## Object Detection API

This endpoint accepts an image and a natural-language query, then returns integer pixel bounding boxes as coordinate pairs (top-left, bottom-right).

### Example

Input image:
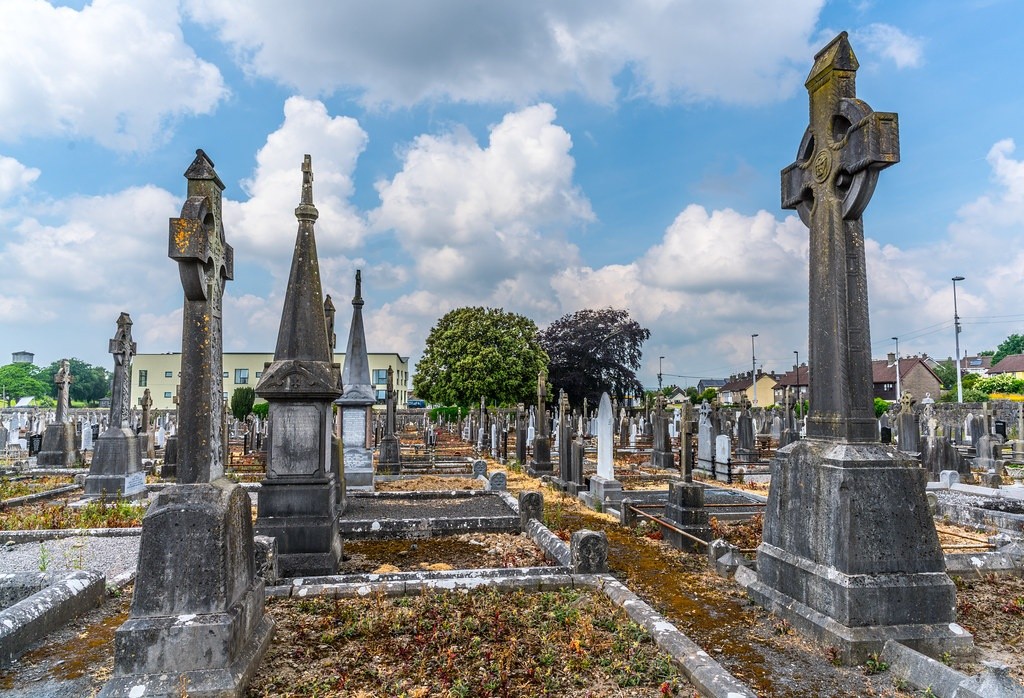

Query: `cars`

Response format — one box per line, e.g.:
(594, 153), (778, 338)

(406, 400), (426, 408)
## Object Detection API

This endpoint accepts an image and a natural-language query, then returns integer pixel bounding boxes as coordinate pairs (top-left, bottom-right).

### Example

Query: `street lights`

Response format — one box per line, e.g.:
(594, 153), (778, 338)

(891, 336), (905, 404)
(751, 333), (761, 407)
(792, 350), (803, 401)
(951, 276), (968, 403)
(656, 355), (664, 389)
